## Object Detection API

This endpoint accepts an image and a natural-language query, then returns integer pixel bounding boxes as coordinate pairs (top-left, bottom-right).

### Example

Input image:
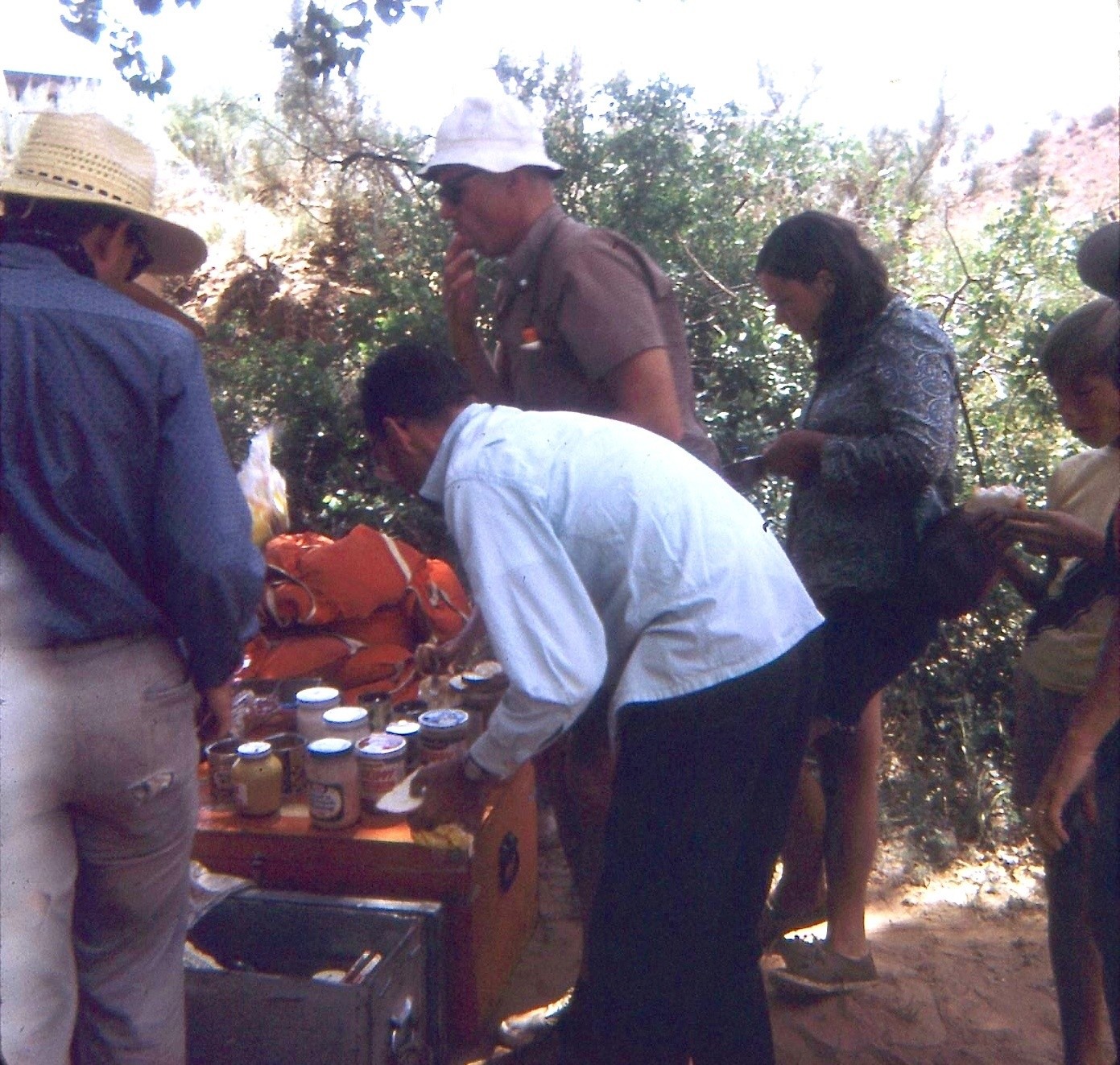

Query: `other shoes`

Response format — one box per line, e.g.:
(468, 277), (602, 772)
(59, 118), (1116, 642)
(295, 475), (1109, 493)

(497, 986), (576, 1049)
(756, 894), (826, 938)
(771, 935), (880, 992)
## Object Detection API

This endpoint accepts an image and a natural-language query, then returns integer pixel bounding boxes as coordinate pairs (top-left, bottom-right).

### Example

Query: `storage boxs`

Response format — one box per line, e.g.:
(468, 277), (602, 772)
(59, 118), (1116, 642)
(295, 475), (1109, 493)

(190, 744), (536, 1044)
(178, 886), (446, 1065)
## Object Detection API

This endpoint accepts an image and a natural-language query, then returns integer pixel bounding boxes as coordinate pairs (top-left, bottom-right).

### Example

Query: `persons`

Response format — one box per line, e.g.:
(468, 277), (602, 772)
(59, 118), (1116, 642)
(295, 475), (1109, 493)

(754, 210), (960, 993)
(1033, 222), (1120, 1051)
(417, 96), (727, 1050)
(970, 296), (1120, 1065)
(0, 110), (265, 1065)
(361, 341), (826, 1065)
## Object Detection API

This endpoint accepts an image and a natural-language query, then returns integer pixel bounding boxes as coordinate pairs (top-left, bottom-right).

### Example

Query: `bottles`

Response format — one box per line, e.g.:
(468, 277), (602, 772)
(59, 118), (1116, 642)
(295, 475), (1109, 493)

(519, 328), (546, 351)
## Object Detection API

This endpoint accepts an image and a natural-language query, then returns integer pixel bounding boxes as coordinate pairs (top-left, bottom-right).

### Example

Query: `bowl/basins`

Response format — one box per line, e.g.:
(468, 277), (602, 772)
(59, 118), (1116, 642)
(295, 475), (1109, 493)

(725, 455), (766, 485)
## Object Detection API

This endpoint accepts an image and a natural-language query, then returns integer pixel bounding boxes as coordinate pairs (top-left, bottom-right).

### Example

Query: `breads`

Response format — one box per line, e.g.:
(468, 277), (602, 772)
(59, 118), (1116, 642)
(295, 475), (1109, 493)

(970, 485), (1027, 508)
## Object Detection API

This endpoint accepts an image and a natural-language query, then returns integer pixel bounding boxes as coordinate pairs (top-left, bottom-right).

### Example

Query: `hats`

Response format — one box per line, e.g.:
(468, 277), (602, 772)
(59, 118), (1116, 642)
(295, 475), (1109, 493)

(416, 93), (563, 178)
(2, 111), (209, 276)
(1077, 222), (1120, 302)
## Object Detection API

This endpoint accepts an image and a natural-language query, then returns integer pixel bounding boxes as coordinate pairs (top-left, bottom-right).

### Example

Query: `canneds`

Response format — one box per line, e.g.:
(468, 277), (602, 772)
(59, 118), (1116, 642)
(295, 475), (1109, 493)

(228, 672), (503, 829)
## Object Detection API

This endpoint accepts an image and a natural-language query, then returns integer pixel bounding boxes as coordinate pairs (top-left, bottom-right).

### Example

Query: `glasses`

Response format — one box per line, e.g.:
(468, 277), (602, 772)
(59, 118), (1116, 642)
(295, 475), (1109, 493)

(131, 233), (154, 278)
(440, 169), (477, 204)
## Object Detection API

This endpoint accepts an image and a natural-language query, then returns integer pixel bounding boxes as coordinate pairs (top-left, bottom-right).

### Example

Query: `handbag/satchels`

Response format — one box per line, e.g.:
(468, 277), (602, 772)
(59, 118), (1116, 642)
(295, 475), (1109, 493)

(900, 504), (1006, 619)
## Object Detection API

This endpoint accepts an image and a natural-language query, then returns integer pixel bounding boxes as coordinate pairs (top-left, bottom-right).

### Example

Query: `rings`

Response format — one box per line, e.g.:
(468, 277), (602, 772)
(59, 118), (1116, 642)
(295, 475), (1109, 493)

(1038, 810), (1045, 814)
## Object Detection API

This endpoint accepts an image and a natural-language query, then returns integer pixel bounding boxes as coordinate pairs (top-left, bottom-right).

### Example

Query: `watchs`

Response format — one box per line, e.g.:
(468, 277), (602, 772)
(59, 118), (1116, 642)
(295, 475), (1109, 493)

(460, 751), (500, 784)
